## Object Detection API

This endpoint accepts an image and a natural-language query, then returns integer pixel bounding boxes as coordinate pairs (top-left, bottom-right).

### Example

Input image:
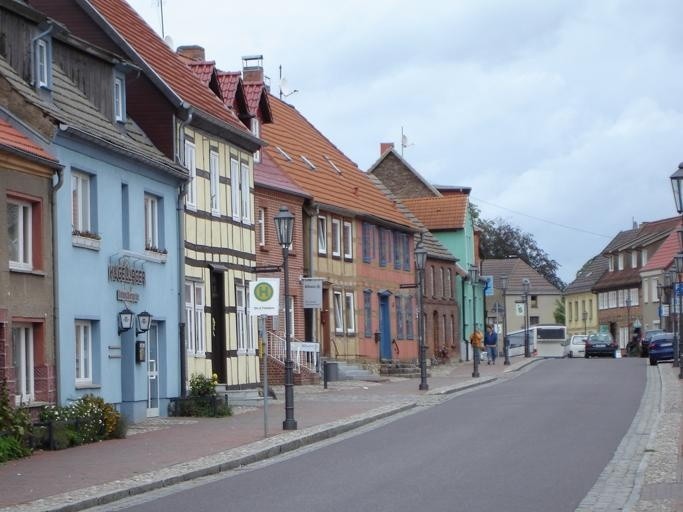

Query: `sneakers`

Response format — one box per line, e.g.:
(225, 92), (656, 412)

(488, 360), (495, 364)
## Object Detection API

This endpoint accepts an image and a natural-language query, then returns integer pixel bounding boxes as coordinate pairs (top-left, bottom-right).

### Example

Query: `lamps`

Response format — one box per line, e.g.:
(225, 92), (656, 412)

(117, 307), (153, 337)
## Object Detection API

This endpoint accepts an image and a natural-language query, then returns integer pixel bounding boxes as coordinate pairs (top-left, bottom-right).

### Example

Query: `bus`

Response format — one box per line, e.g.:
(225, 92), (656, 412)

(505, 325), (567, 359)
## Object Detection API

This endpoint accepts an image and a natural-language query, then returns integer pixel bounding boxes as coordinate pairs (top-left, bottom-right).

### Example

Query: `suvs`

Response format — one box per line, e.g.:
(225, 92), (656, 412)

(585, 333), (615, 358)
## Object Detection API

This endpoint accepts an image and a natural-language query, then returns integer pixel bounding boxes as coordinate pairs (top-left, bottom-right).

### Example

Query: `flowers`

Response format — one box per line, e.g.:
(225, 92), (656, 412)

(180, 373), (219, 397)
(39, 393), (121, 449)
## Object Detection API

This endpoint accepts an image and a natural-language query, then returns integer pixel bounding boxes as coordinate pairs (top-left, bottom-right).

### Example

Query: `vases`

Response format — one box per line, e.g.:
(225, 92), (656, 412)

(29, 419), (82, 451)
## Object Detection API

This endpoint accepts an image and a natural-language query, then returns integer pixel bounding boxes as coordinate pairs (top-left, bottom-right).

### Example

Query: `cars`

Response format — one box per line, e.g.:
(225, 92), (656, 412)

(566, 335), (594, 358)
(640, 329), (680, 365)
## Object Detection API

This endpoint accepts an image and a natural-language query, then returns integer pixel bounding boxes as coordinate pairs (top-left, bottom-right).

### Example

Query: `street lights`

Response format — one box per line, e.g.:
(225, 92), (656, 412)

(656, 282), (664, 329)
(500, 273), (510, 366)
(274, 207), (297, 430)
(626, 297), (632, 353)
(467, 264), (480, 378)
(664, 162), (683, 379)
(522, 278), (530, 357)
(413, 243), (429, 391)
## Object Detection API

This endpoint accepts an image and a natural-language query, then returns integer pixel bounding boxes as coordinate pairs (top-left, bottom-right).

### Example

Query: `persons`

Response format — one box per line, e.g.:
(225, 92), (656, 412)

(469, 328), (482, 363)
(484, 325), (498, 364)
